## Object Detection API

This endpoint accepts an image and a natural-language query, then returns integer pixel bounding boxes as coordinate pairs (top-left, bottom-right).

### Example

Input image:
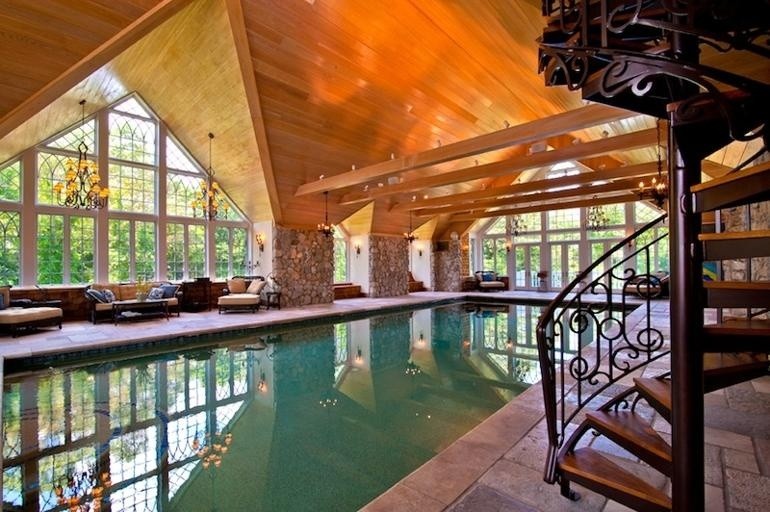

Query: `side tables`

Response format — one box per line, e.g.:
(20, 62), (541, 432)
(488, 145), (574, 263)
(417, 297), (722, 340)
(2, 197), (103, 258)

(268, 292), (281, 310)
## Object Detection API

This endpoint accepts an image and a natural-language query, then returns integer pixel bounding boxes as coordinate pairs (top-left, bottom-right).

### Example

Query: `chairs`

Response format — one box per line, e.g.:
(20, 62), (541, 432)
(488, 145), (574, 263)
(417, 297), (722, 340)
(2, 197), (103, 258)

(475, 271), (505, 291)
(624, 275), (670, 298)
(0, 285), (63, 337)
(218, 275), (267, 313)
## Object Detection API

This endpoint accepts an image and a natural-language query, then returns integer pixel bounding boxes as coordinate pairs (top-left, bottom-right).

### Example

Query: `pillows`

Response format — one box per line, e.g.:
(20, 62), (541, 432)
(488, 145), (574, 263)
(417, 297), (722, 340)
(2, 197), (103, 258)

(476, 272), (497, 280)
(86, 287), (115, 304)
(226, 278), (266, 294)
(150, 283), (182, 299)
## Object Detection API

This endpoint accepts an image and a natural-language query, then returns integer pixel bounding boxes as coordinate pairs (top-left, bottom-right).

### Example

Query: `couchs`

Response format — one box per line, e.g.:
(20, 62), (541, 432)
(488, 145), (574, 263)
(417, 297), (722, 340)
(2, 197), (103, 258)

(85, 283), (187, 324)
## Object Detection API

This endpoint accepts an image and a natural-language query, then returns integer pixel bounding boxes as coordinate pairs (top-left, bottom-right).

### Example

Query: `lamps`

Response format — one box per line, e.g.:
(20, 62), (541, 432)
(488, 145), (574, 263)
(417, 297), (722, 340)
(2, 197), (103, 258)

(638, 117), (670, 210)
(317, 191), (337, 238)
(190, 130), (230, 222)
(53, 95), (109, 209)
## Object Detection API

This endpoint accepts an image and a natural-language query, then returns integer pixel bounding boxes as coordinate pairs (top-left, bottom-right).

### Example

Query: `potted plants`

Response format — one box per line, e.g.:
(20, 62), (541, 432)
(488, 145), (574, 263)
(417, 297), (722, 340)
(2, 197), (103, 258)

(135, 283), (149, 300)
